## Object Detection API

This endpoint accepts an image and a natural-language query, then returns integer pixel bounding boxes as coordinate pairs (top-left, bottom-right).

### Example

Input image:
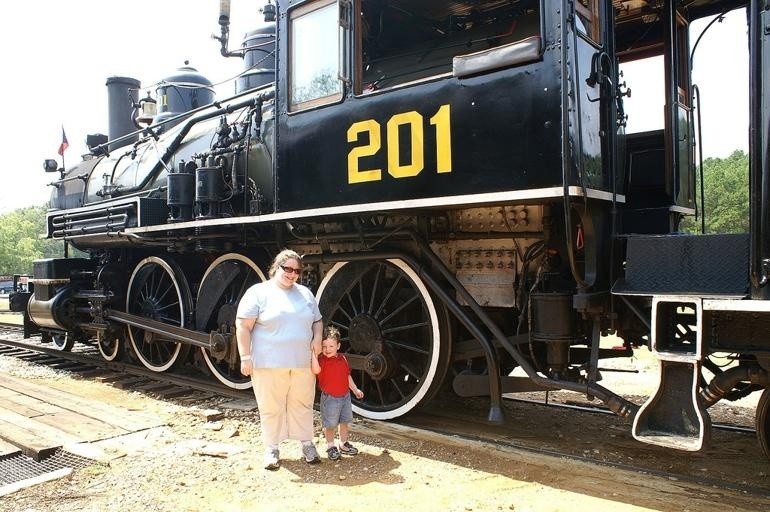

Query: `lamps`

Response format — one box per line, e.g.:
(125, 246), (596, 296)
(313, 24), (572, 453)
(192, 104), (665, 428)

(126, 89), (157, 136)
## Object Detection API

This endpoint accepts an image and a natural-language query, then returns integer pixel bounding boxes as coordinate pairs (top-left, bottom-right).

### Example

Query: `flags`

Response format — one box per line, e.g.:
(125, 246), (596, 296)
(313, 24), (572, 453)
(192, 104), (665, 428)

(57, 126), (71, 157)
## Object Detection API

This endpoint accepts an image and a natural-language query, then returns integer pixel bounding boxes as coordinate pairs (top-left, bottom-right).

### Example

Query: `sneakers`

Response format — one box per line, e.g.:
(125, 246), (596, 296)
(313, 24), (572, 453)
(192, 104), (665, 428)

(326, 446), (343, 461)
(339, 442), (359, 456)
(302, 443), (321, 464)
(264, 451), (280, 470)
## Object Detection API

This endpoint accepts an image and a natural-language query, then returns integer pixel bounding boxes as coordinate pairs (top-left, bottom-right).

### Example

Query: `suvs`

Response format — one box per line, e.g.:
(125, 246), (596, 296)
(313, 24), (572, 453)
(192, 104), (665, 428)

(0, 281), (14, 294)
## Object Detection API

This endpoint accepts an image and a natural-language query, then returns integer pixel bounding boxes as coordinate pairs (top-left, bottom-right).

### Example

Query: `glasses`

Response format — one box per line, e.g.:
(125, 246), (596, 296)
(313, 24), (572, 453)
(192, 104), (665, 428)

(279, 265), (302, 275)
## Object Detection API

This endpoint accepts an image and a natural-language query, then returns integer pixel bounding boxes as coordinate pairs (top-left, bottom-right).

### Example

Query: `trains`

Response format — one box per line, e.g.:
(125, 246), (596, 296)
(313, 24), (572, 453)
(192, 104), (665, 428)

(9, 1), (770, 462)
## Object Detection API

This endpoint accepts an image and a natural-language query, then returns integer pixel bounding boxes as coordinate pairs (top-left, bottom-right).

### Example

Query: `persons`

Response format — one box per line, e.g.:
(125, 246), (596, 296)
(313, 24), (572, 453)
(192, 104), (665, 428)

(233, 248), (325, 471)
(310, 322), (366, 462)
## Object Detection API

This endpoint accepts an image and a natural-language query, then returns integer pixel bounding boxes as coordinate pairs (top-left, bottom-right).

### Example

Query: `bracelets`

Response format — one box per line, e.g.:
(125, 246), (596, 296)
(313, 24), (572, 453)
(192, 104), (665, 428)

(239, 354), (252, 362)
